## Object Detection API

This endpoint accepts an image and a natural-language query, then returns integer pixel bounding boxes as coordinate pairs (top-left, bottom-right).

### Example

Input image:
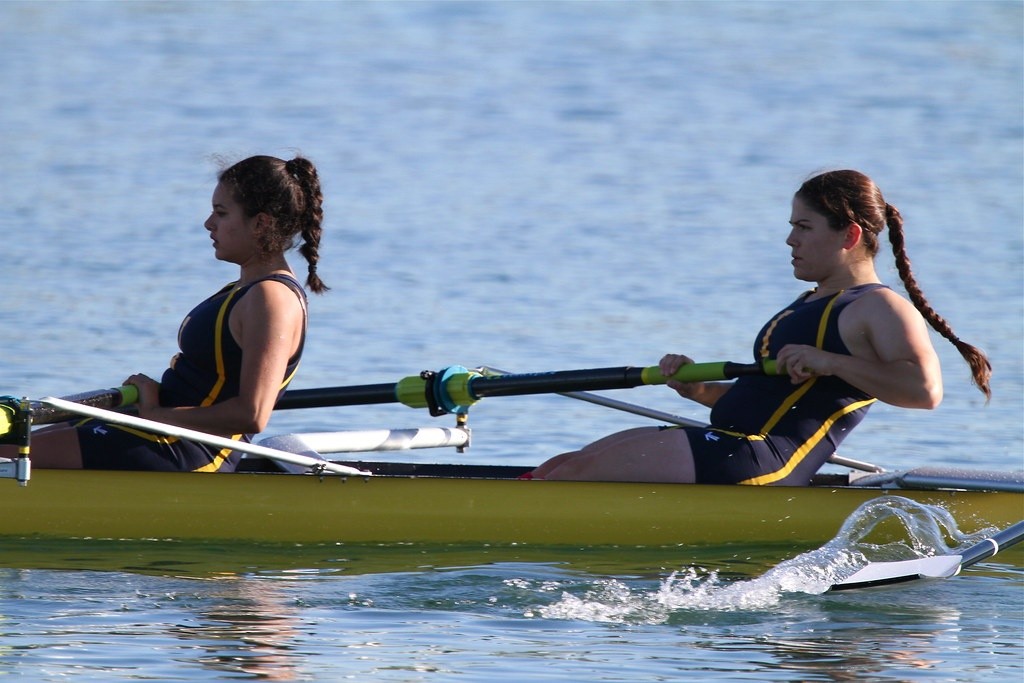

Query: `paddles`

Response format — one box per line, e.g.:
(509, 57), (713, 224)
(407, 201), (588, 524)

(0, 382), (140, 489)
(242, 422), (473, 477)
(825, 518), (1023, 592)
(271, 355), (805, 413)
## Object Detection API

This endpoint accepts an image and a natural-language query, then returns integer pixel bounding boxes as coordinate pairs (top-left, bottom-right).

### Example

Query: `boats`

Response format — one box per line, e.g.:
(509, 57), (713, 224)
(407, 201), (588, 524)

(0, 426), (1024, 574)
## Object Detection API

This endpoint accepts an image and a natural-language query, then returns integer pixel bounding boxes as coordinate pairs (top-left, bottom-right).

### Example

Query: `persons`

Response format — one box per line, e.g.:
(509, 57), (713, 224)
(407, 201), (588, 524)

(517, 169), (994, 489)
(0, 154), (330, 473)
